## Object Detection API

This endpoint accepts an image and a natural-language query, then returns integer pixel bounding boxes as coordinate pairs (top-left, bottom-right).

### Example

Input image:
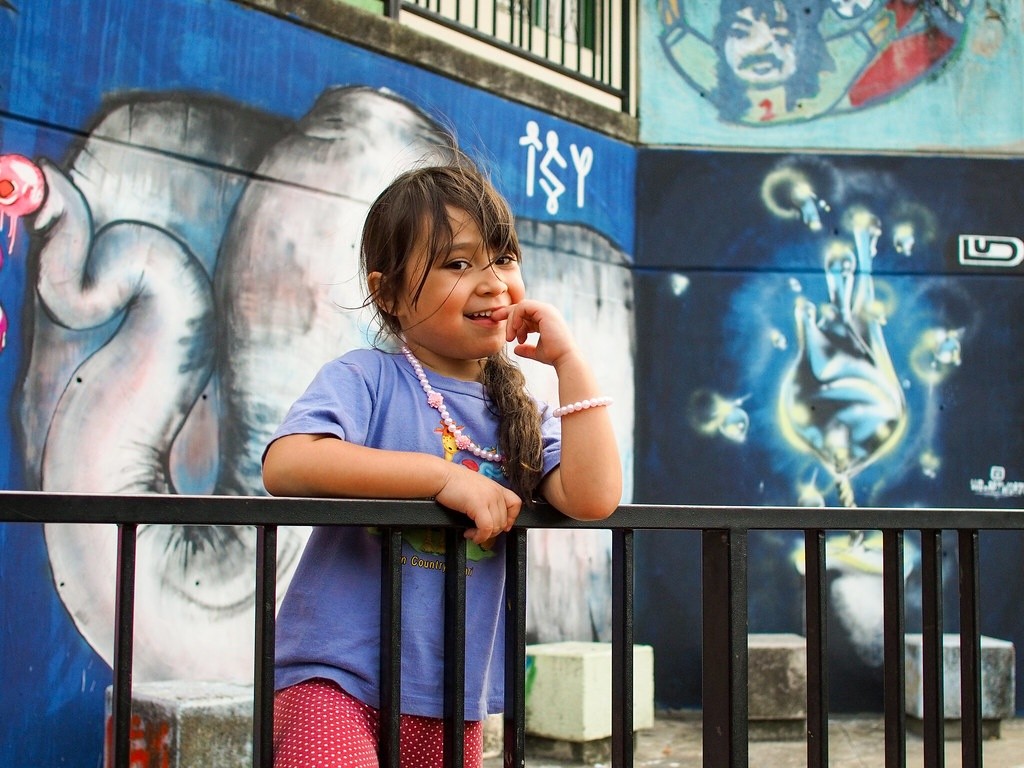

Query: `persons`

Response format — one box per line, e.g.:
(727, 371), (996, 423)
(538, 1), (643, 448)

(265, 164), (623, 768)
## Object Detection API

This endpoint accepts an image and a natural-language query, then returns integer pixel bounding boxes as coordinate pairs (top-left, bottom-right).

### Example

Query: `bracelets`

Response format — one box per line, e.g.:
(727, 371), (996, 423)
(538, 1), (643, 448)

(554, 396), (616, 417)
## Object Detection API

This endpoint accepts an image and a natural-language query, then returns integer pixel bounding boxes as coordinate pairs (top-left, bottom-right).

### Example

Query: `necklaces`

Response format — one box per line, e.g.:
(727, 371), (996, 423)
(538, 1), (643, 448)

(401, 348), (508, 462)
